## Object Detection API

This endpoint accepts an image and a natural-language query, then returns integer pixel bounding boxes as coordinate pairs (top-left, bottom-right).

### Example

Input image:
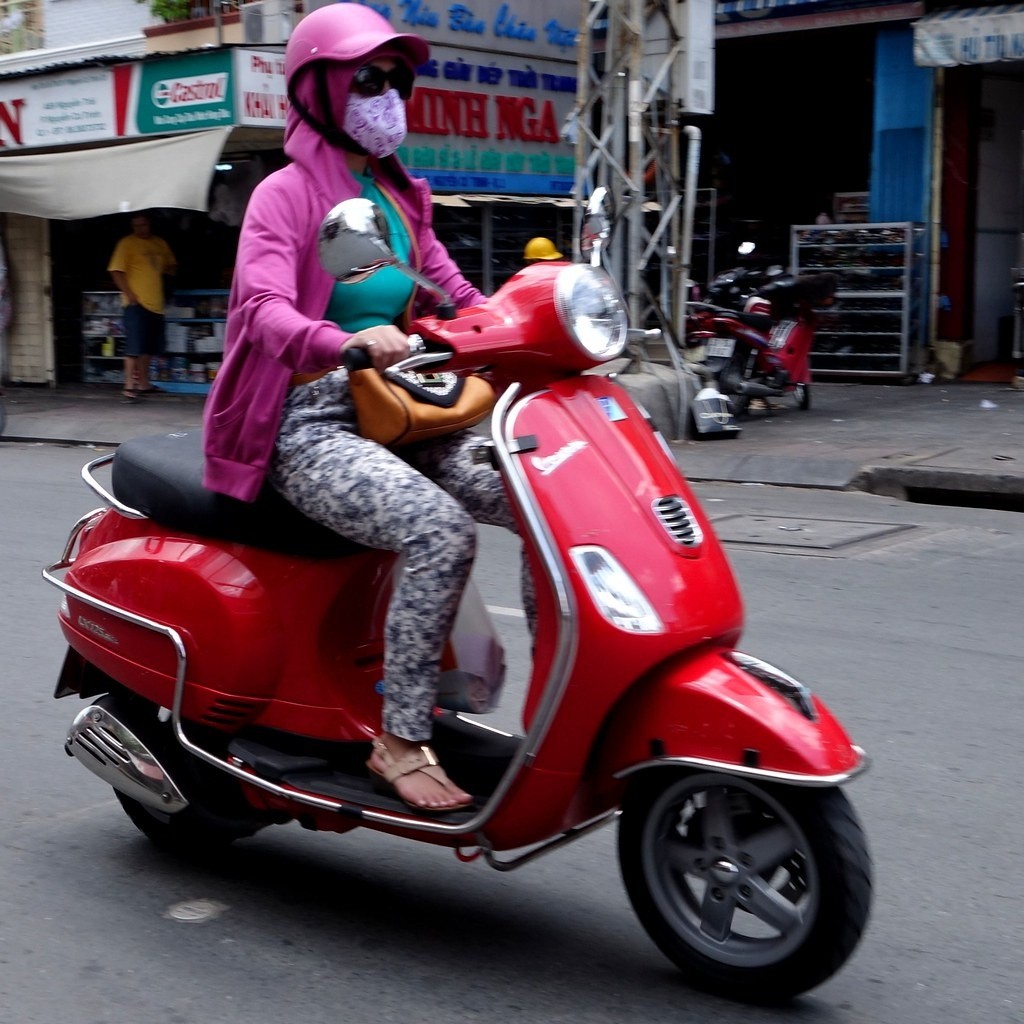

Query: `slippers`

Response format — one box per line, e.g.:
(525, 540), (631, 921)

(118, 389), (143, 404)
(137, 385), (167, 393)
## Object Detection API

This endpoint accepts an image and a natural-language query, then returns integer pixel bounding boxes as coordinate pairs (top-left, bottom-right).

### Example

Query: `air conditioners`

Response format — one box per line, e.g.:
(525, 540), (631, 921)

(240, 1), (290, 44)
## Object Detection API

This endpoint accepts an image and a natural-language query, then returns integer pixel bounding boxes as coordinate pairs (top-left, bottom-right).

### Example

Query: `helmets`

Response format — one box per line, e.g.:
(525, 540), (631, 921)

(284, 2), (430, 156)
(523, 237), (564, 261)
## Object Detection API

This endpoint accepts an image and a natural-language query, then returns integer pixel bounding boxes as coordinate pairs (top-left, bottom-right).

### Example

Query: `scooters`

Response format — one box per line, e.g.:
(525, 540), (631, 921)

(44, 185), (873, 1007)
(685, 239), (840, 418)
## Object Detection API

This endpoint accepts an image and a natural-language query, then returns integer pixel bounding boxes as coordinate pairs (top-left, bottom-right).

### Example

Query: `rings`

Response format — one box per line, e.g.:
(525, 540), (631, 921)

(366, 340), (378, 347)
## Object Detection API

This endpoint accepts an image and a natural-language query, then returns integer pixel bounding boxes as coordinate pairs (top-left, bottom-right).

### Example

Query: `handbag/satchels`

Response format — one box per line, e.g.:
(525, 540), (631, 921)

(347, 178), (498, 448)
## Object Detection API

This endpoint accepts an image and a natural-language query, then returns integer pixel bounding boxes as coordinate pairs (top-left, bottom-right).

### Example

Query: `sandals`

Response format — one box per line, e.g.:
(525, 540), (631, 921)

(365, 737), (477, 814)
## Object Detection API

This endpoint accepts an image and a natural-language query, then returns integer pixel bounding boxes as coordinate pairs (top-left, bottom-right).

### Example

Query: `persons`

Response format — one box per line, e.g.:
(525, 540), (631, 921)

(372, 206), (391, 253)
(107, 211), (178, 403)
(522, 236), (565, 267)
(204, 2), (537, 814)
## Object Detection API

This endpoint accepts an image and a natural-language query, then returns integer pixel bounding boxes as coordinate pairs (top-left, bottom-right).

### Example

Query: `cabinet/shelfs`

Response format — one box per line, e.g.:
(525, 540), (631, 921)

(80, 289), (228, 394)
(643, 188), (721, 298)
(792, 223), (927, 387)
(432, 202), (558, 294)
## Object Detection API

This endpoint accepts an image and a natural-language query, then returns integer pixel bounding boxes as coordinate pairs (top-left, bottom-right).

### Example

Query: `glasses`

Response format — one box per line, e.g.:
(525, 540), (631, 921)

(350, 63), (415, 100)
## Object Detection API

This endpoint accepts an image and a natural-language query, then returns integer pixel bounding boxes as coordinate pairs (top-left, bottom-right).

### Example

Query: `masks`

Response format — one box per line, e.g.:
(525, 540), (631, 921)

(343, 88), (408, 159)
